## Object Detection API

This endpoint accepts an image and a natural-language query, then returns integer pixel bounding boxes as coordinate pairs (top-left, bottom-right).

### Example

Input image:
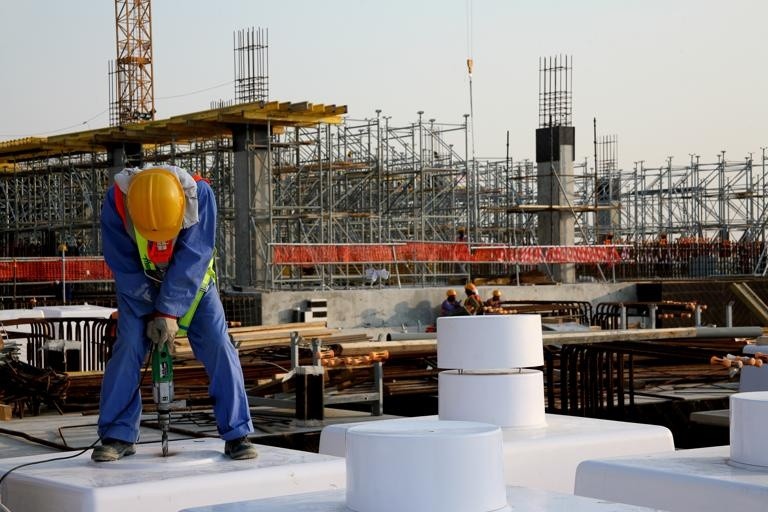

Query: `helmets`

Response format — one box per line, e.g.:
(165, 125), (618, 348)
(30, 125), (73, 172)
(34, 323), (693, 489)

(445, 280), (502, 297)
(125, 167), (189, 242)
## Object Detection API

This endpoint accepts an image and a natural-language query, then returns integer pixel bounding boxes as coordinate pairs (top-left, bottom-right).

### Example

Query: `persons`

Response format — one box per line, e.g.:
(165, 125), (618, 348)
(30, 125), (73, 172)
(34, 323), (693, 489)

(655, 232), (668, 248)
(90, 159), (257, 463)
(485, 289), (502, 313)
(600, 230), (616, 246)
(455, 228), (467, 241)
(441, 287), (463, 316)
(463, 284), (484, 316)
(52, 243), (75, 305)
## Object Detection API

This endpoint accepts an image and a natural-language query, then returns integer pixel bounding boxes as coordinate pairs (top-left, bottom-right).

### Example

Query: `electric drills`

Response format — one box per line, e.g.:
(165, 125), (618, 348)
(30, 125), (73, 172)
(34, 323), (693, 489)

(151, 329), (174, 457)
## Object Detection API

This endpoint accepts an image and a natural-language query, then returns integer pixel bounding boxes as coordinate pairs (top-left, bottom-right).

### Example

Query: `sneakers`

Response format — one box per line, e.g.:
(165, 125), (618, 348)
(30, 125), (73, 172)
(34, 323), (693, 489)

(221, 435), (257, 461)
(88, 437), (136, 464)
